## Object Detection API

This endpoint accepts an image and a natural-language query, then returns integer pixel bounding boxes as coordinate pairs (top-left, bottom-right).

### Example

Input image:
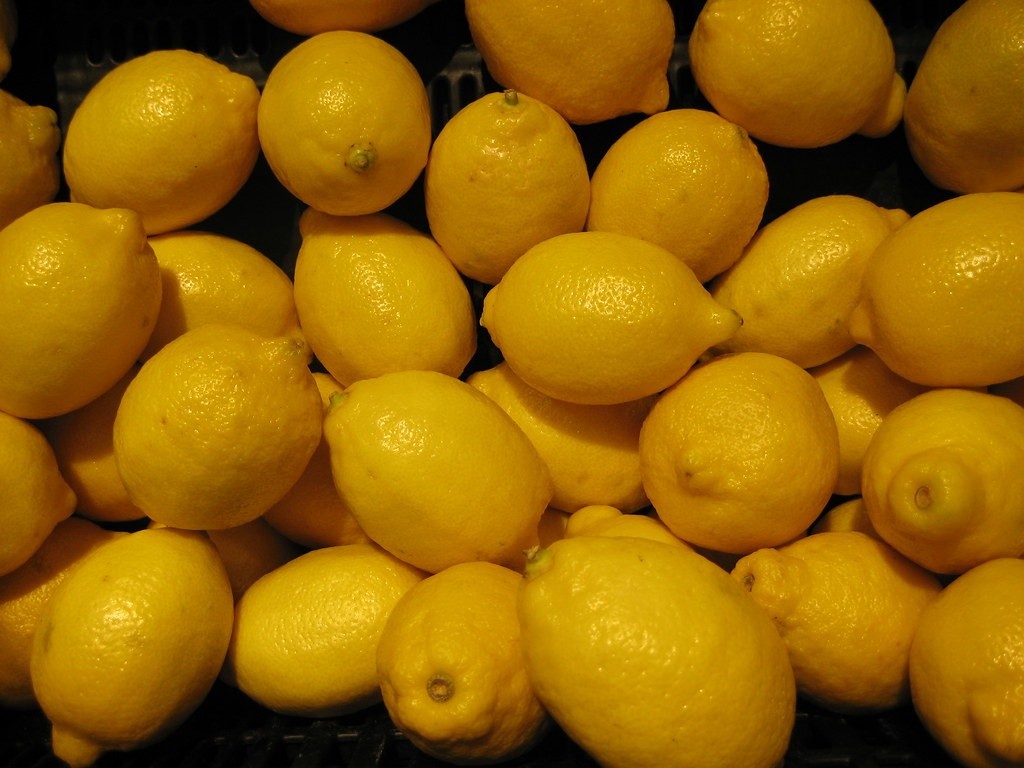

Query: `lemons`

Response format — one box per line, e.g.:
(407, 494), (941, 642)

(0, 0), (1024, 768)
